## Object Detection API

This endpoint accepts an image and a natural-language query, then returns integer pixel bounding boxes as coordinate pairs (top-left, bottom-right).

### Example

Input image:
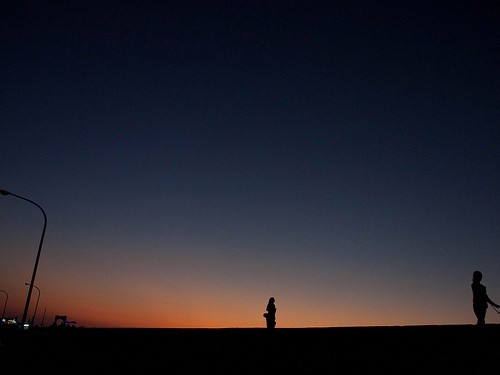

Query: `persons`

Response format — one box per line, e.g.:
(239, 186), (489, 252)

(263, 296), (277, 328)
(471, 271), (500, 324)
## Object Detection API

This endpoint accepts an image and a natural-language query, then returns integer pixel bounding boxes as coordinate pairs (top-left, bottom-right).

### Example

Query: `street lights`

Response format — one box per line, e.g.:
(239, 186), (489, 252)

(25, 283), (47, 327)
(0, 189), (47, 328)
